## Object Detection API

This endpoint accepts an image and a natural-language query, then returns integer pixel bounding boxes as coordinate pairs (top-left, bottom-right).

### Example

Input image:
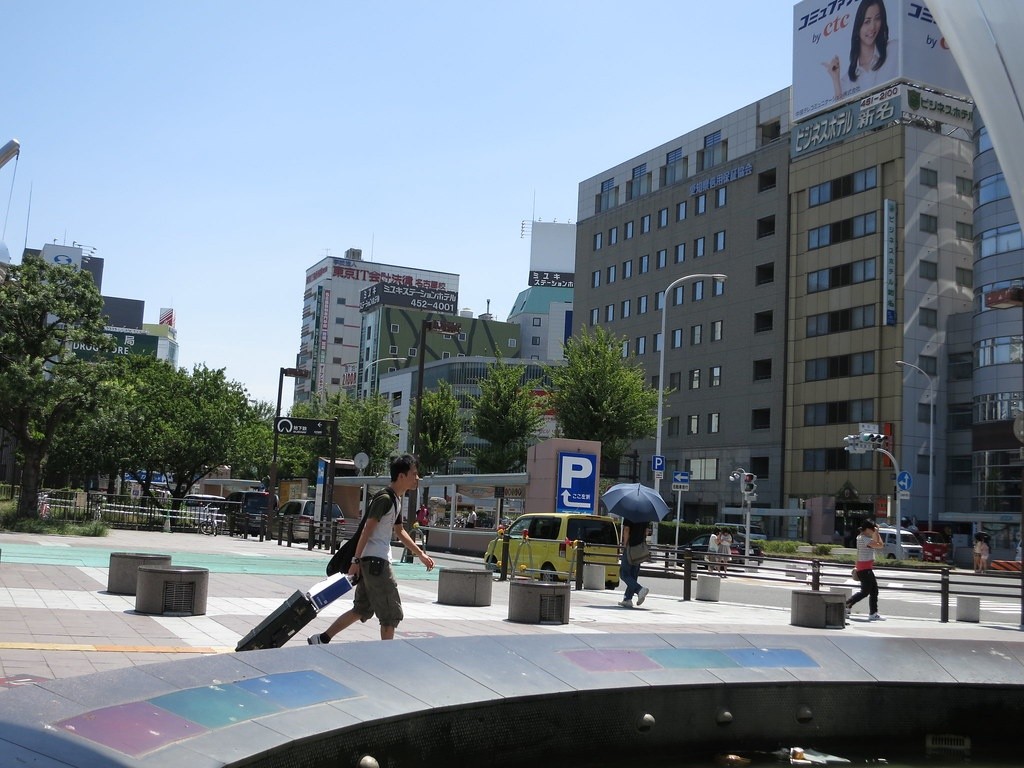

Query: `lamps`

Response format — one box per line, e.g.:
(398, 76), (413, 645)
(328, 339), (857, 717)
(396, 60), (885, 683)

(53, 238), (97, 264)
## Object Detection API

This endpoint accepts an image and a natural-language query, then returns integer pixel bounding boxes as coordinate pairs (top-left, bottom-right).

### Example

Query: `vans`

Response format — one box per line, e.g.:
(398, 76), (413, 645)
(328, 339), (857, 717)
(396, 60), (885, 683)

(909, 531), (948, 562)
(714, 523), (768, 541)
(484, 512), (621, 589)
(874, 527), (923, 560)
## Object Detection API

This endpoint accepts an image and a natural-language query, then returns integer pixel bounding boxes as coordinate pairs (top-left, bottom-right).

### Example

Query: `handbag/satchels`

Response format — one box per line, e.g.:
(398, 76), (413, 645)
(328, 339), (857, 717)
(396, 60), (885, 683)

(852, 569), (860, 581)
(326, 533), (362, 586)
(306, 572), (354, 610)
(627, 541), (651, 565)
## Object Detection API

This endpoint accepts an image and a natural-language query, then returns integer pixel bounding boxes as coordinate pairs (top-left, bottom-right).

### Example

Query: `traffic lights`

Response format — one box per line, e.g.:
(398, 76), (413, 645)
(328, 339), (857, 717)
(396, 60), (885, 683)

(844, 445), (866, 453)
(740, 473), (757, 493)
(843, 435), (858, 444)
(859, 432), (889, 445)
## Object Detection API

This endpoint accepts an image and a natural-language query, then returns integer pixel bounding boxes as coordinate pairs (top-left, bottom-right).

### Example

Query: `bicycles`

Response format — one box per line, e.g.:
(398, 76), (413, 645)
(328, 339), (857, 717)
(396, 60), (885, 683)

(199, 502), (218, 537)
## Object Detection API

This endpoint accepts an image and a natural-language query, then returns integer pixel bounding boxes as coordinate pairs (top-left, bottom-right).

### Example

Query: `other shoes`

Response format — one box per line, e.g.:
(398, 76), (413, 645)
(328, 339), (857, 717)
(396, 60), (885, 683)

(307, 634), (325, 644)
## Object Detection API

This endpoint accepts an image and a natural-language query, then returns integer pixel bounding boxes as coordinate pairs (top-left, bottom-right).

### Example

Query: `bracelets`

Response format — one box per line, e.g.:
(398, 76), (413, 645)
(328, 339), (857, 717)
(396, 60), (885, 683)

(351, 557), (360, 564)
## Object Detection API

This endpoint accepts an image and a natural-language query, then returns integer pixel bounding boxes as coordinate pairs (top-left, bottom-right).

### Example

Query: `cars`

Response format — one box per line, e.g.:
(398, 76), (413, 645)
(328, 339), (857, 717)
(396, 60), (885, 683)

(671, 534), (761, 569)
(263, 499), (347, 550)
(182, 494), (226, 525)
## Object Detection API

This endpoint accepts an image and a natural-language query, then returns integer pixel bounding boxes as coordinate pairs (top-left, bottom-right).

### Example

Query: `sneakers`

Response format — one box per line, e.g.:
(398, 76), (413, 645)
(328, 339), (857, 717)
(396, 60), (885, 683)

(618, 599), (633, 607)
(637, 587), (649, 605)
(869, 613), (887, 621)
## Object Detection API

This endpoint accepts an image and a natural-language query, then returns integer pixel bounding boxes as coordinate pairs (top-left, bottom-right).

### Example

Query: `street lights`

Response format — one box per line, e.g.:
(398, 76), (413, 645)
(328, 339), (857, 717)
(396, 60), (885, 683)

(654, 273), (728, 494)
(265, 367), (311, 541)
(360, 357), (408, 406)
(895, 360), (934, 531)
(404, 318), (462, 564)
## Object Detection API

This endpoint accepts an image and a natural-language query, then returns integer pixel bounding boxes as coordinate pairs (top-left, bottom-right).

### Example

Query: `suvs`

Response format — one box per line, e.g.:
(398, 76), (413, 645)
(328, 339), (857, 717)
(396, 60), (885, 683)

(218, 491), (280, 537)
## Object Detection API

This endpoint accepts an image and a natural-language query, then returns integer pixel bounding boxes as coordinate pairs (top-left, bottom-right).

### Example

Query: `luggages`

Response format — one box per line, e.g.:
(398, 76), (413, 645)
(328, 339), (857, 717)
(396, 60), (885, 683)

(235, 576), (360, 652)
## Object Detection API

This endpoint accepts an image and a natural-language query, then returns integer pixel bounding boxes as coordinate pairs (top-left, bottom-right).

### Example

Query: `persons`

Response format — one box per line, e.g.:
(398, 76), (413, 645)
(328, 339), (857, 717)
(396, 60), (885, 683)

(307, 453), (435, 645)
(973, 536), (989, 573)
(618, 518), (649, 607)
(465, 506), (477, 528)
(822, 0), (899, 101)
(846, 519), (887, 620)
(707, 527), (732, 577)
(416, 504), (429, 526)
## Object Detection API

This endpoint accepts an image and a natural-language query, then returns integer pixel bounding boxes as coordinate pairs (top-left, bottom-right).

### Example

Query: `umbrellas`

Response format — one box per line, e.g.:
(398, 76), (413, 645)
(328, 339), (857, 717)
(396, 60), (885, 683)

(974, 531), (991, 541)
(600, 482), (672, 523)
